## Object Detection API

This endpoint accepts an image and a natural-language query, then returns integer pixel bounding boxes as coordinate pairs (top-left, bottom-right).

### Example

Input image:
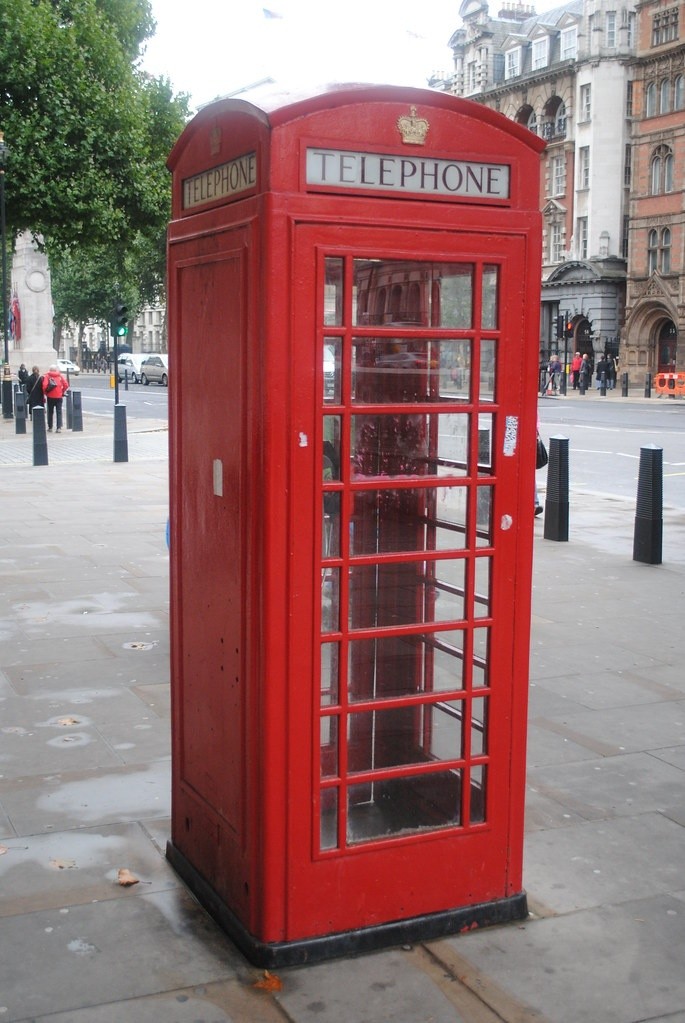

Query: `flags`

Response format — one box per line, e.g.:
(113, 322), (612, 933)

(9, 296), (20, 340)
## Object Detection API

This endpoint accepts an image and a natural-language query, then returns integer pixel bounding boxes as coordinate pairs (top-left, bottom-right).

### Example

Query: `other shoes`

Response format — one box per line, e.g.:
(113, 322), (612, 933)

(596, 387), (600, 390)
(606, 387), (609, 390)
(534, 505), (543, 515)
(55, 429), (61, 433)
(609, 387), (612, 390)
(571, 387), (575, 390)
(46, 429), (51, 432)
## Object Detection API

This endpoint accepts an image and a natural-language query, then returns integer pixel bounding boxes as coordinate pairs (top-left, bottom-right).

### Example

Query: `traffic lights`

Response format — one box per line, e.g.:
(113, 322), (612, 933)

(553, 314), (563, 340)
(566, 322), (574, 340)
(112, 306), (128, 336)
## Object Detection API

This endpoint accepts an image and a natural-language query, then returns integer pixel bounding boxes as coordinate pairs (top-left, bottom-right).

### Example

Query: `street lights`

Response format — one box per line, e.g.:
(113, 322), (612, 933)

(0, 130), (12, 418)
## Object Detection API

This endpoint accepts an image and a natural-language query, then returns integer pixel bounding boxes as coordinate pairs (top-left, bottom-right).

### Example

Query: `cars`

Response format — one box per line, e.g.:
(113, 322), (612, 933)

(140, 354), (168, 387)
(117, 353), (148, 383)
(57, 358), (80, 376)
(323, 346), (336, 390)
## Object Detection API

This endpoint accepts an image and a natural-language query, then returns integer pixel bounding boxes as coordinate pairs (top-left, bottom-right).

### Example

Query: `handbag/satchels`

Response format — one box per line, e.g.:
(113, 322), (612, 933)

(569, 371), (574, 383)
(535, 429), (548, 470)
(614, 366), (620, 372)
(25, 394), (30, 404)
(45, 377), (56, 392)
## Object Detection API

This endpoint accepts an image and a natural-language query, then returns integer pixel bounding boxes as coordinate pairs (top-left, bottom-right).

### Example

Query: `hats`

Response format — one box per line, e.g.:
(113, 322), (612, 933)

(575, 352), (580, 355)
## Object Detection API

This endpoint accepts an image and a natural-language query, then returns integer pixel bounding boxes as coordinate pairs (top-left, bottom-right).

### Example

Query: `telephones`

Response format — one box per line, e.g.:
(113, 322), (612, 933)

(323, 439), (341, 513)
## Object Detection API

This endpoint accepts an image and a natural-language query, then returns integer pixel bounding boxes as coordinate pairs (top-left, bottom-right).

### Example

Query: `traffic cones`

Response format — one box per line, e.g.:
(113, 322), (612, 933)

(546, 376), (555, 395)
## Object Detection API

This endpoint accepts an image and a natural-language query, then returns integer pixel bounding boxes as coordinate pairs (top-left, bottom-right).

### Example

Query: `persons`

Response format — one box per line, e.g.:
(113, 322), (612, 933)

(18, 364), (68, 433)
(549, 352), (617, 395)
